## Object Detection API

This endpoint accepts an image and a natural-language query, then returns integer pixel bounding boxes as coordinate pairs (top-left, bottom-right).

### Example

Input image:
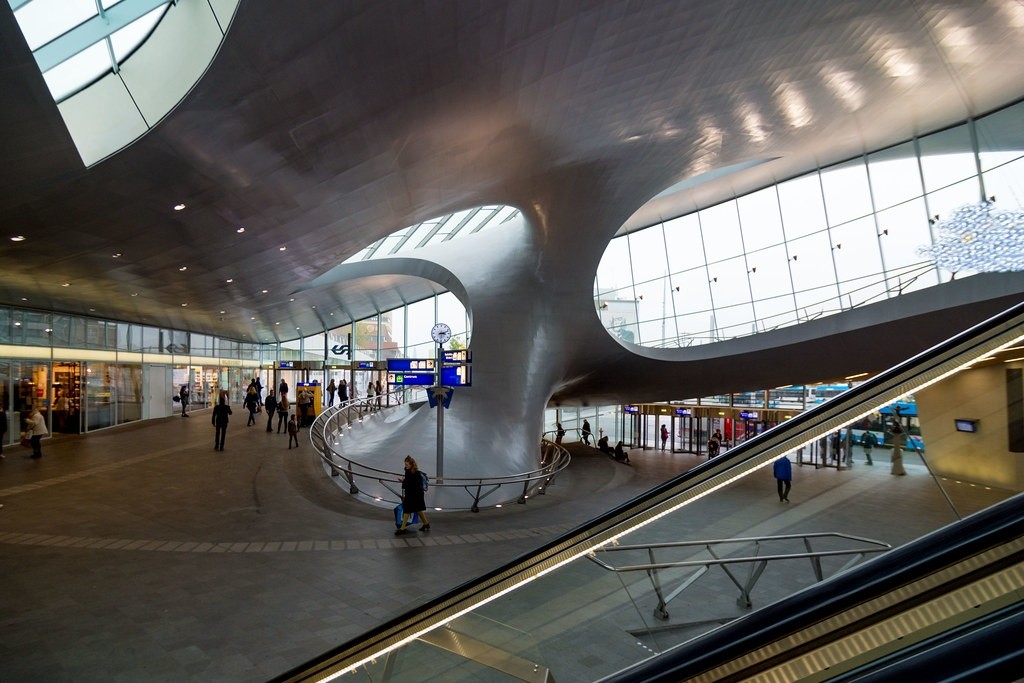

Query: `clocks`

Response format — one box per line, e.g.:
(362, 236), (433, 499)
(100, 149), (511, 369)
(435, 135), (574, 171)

(431, 323), (451, 343)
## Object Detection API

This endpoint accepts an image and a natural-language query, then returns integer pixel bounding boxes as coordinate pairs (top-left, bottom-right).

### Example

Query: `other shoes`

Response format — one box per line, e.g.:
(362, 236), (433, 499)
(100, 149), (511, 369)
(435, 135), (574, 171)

(783, 496), (789, 501)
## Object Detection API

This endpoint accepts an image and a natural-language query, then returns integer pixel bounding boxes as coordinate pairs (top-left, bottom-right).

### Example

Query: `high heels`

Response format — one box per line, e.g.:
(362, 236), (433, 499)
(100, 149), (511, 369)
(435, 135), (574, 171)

(419, 525), (430, 531)
(395, 530), (406, 535)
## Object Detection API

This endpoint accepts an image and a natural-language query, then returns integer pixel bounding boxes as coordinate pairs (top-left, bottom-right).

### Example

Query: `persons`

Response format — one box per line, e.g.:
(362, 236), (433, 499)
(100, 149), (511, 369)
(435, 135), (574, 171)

(54, 385), (66, 406)
(0, 403), (7, 508)
(180, 385), (191, 418)
(24, 409), (48, 459)
(212, 394), (232, 451)
(398, 455), (428, 535)
(555, 419), (874, 502)
(243, 376), (384, 451)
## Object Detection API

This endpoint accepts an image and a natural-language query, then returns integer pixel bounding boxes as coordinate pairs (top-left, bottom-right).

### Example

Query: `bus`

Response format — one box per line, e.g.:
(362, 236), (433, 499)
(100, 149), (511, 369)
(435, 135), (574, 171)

(719, 385), (855, 413)
(833, 402), (924, 451)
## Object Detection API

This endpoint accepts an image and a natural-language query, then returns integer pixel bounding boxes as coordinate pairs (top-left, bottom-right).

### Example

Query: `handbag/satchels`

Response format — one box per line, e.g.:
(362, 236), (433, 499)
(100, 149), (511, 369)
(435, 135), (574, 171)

(394, 502), (419, 527)
(25, 429), (33, 440)
(419, 471), (429, 491)
